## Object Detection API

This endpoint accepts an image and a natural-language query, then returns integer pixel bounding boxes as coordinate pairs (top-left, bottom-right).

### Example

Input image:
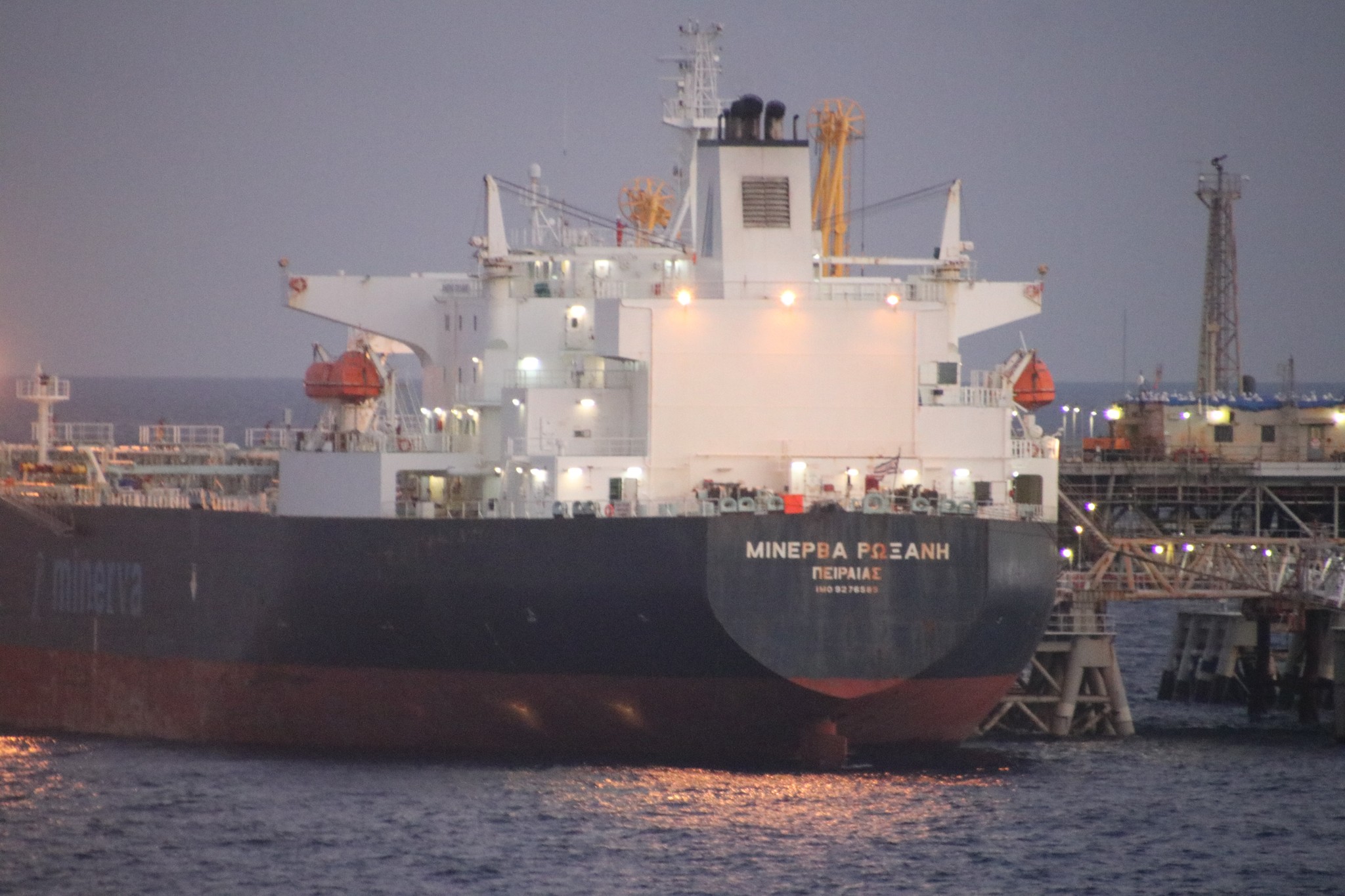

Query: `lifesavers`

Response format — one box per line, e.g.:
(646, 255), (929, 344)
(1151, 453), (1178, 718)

(719, 494), (786, 514)
(398, 439), (412, 452)
(862, 494), (977, 516)
(293, 279), (308, 292)
(1027, 442), (1039, 458)
(603, 505), (615, 516)
